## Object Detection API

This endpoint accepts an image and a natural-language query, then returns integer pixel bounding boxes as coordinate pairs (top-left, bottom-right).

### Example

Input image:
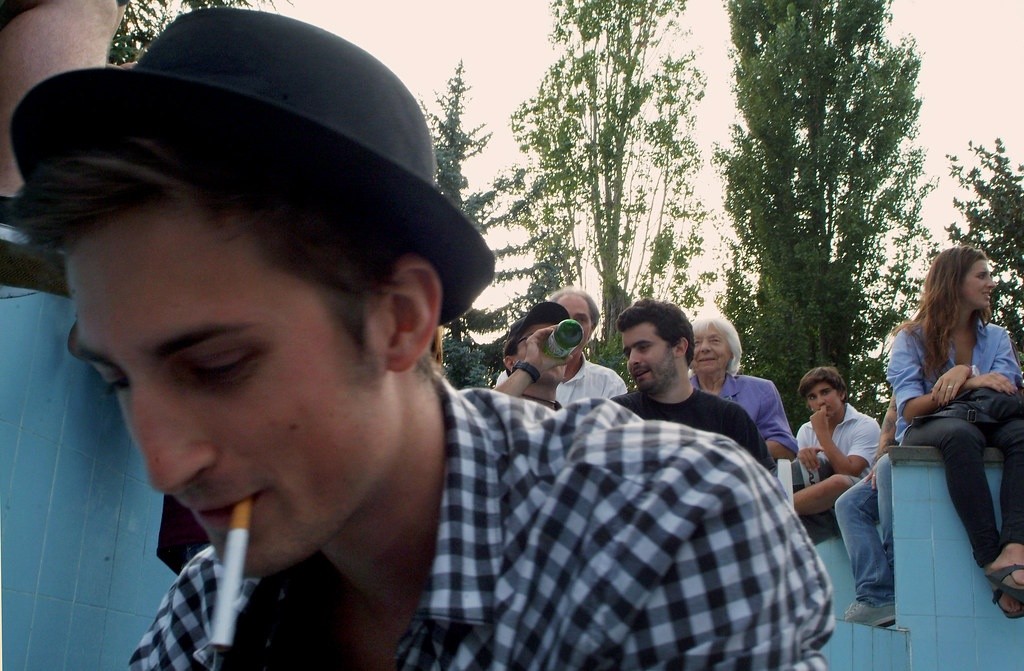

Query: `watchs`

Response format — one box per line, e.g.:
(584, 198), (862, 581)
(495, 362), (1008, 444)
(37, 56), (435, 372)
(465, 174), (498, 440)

(970, 363), (979, 378)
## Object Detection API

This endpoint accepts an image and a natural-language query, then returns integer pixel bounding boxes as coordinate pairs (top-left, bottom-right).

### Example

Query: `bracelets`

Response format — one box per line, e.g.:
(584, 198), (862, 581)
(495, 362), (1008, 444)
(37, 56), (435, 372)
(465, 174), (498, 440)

(965, 363), (972, 379)
(510, 360), (540, 383)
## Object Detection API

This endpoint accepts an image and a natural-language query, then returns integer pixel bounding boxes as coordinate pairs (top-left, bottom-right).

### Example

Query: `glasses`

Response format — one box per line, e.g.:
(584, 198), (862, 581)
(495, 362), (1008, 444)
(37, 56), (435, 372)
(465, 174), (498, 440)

(514, 334), (532, 345)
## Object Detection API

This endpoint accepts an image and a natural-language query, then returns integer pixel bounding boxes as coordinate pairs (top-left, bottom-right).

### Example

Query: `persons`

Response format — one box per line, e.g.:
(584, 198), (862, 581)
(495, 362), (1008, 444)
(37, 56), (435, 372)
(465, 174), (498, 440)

(835, 391), (898, 626)
(9, 10), (836, 671)
(488, 286), (626, 413)
(792, 366), (882, 516)
(885, 246), (1024, 618)
(688, 314), (800, 463)
(609, 298), (779, 478)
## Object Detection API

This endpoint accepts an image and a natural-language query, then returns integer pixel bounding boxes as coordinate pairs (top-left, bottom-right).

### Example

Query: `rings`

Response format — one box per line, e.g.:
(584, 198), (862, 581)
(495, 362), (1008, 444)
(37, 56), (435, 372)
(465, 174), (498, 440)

(873, 474), (876, 476)
(948, 386), (953, 389)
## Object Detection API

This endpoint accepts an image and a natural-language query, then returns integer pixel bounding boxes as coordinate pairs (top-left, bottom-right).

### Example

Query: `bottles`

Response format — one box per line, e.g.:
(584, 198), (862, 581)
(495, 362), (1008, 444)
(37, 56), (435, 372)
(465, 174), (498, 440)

(541, 319), (587, 360)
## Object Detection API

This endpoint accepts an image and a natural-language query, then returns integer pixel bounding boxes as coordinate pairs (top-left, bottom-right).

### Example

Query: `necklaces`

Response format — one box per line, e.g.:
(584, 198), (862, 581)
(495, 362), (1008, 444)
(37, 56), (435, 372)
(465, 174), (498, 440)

(522, 394), (556, 404)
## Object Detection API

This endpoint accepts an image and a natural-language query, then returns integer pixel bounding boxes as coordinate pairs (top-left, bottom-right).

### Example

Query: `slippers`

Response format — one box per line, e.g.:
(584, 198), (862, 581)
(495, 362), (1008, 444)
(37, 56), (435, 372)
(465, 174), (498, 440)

(984, 564), (1024, 619)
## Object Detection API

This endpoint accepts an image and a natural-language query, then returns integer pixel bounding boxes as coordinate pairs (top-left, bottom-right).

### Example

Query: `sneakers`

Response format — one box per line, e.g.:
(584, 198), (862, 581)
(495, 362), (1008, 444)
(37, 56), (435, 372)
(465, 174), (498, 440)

(844, 601), (895, 627)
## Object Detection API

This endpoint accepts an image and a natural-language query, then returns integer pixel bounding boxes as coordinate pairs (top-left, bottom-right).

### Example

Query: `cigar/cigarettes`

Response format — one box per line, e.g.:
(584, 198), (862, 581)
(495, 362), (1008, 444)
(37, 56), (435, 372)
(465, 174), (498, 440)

(210, 496), (254, 651)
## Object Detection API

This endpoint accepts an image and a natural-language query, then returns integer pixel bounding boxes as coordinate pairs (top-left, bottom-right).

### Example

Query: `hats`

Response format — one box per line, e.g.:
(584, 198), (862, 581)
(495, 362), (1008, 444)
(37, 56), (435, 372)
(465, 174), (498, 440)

(10, 8), (497, 327)
(502, 302), (570, 347)
